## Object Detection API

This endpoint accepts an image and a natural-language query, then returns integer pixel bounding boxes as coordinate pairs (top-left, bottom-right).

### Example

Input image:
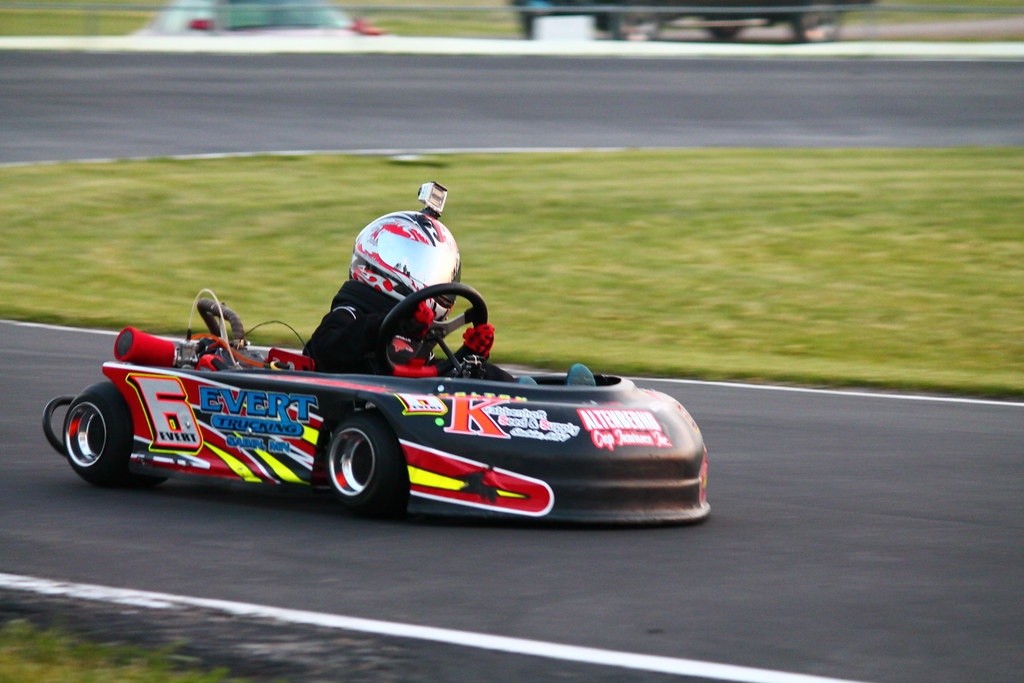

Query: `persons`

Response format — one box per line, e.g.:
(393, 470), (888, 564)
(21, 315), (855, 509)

(303, 211), (596, 385)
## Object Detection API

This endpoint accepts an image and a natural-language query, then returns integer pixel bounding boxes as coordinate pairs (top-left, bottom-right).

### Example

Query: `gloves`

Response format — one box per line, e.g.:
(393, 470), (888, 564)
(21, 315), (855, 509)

(416, 301), (436, 336)
(464, 323), (495, 358)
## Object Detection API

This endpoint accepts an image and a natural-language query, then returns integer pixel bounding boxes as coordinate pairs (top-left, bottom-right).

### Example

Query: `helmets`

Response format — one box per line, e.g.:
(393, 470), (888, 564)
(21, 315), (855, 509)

(348, 210), (461, 327)
